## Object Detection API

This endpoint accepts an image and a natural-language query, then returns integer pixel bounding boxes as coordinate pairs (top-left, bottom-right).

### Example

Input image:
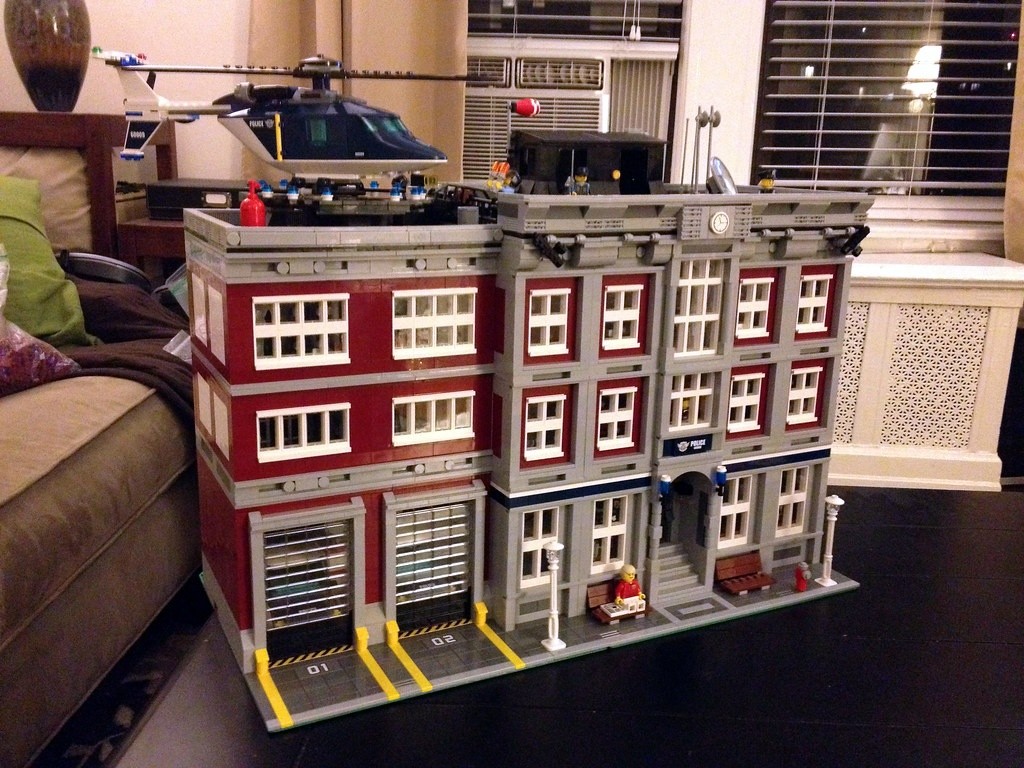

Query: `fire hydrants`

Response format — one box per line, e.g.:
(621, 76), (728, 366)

(794, 561), (813, 594)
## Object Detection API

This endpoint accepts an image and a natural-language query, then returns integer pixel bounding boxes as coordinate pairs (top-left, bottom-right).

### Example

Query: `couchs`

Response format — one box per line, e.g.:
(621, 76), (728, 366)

(0, 329), (203, 768)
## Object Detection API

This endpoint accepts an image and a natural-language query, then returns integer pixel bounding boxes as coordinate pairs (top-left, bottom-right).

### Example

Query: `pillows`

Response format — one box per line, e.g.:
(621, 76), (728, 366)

(0, 176), (103, 347)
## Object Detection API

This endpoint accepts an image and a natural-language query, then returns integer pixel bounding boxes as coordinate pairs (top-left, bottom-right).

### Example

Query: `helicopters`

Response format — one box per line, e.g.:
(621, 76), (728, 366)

(121, 53), (507, 198)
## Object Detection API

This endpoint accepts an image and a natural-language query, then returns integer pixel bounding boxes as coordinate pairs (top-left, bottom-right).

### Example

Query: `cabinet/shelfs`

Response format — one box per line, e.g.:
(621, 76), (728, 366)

(827, 253), (1024, 493)
(117, 220), (187, 271)
(1, 110), (178, 261)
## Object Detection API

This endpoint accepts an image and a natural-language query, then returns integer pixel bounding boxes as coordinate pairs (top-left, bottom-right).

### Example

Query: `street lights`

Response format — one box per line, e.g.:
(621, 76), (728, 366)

(542, 538), (566, 654)
(813, 495), (846, 589)
